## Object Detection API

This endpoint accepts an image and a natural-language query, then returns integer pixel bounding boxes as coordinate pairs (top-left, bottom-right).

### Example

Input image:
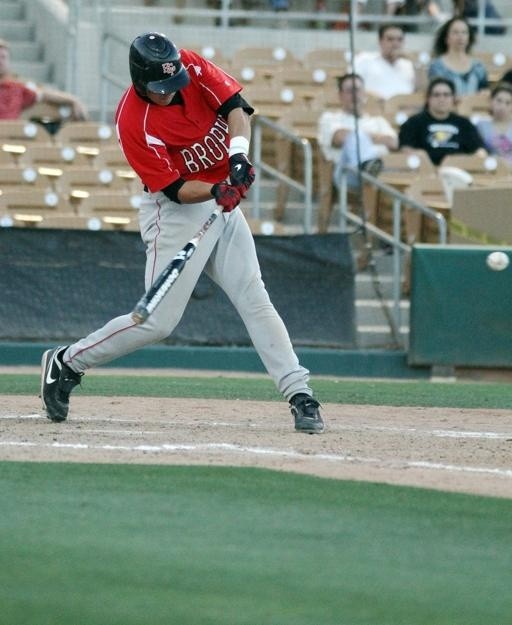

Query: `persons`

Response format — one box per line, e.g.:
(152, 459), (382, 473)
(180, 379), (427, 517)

(317, 0), (512, 211)
(213, 1), (294, 29)
(0, 39), (90, 121)
(42, 31), (325, 434)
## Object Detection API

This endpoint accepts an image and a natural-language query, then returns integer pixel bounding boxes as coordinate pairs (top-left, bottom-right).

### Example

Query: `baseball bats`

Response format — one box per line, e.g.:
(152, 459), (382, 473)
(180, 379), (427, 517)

(132, 205), (225, 326)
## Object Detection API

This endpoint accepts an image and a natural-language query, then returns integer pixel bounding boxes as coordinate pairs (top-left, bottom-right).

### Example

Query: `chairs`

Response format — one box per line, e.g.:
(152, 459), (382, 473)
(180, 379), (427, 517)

(0, 48), (512, 299)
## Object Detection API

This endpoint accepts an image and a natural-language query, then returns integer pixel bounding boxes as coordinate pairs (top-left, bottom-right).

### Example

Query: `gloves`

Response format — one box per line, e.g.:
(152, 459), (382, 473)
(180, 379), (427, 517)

(211, 183), (241, 213)
(228, 137), (255, 195)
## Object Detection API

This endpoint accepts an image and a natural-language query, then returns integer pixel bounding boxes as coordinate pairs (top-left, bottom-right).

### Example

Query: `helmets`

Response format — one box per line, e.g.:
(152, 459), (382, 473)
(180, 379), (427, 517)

(130, 33), (190, 97)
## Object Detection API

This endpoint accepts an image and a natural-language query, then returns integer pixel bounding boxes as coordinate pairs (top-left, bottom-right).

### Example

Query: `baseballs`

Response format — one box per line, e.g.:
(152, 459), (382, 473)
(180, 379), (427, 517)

(487, 252), (509, 270)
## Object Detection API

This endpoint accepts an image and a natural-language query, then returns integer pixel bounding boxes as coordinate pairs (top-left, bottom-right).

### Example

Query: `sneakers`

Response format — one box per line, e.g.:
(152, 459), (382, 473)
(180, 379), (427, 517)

(365, 158), (382, 177)
(291, 396), (325, 434)
(42, 346), (81, 424)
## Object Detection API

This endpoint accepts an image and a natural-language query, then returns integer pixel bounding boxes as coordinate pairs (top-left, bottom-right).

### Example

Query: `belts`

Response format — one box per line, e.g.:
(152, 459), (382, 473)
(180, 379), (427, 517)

(143, 185), (149, 193)
